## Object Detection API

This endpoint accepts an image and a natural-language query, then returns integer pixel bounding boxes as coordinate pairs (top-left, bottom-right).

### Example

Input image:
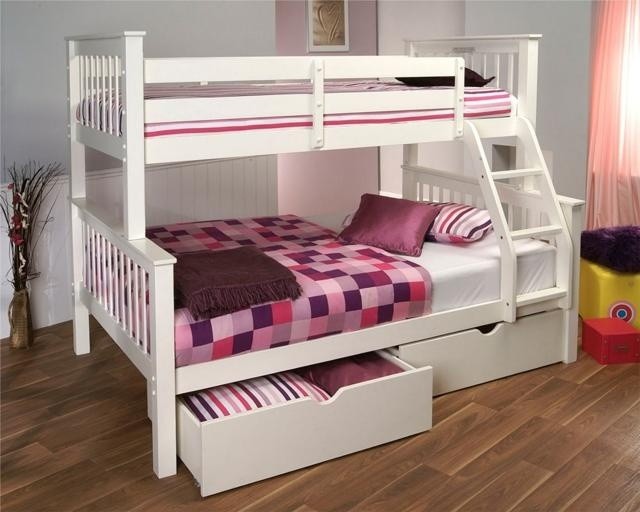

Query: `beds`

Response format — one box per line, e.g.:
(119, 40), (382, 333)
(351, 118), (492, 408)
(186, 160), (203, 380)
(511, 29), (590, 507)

(64, 30), (588, 501)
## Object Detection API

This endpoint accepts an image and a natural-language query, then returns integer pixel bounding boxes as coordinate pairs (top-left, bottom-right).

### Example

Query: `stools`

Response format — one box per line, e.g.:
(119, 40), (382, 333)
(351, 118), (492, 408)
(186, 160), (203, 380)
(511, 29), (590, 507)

(578, 256), (640, 329)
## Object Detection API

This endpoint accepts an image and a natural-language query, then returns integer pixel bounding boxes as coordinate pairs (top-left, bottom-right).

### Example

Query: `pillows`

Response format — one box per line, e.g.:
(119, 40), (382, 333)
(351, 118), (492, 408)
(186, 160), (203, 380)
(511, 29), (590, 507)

(394, 65), (496, 87)
(179, 351), (405, 424)
(334, 191), (493, 258)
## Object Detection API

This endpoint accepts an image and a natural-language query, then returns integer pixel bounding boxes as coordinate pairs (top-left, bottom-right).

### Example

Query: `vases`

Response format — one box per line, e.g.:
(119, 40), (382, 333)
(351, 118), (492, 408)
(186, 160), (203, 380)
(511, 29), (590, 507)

(7, 288), (33, 350)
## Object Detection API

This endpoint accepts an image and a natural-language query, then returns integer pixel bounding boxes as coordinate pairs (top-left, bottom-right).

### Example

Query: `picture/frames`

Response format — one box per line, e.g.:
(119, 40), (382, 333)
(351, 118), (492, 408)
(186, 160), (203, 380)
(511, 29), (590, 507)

(305, 0), (350, 53)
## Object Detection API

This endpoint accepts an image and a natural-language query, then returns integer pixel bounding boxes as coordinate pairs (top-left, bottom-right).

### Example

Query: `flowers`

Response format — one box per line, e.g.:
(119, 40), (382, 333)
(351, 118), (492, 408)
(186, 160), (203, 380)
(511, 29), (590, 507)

(0, 153), (66, 291)
